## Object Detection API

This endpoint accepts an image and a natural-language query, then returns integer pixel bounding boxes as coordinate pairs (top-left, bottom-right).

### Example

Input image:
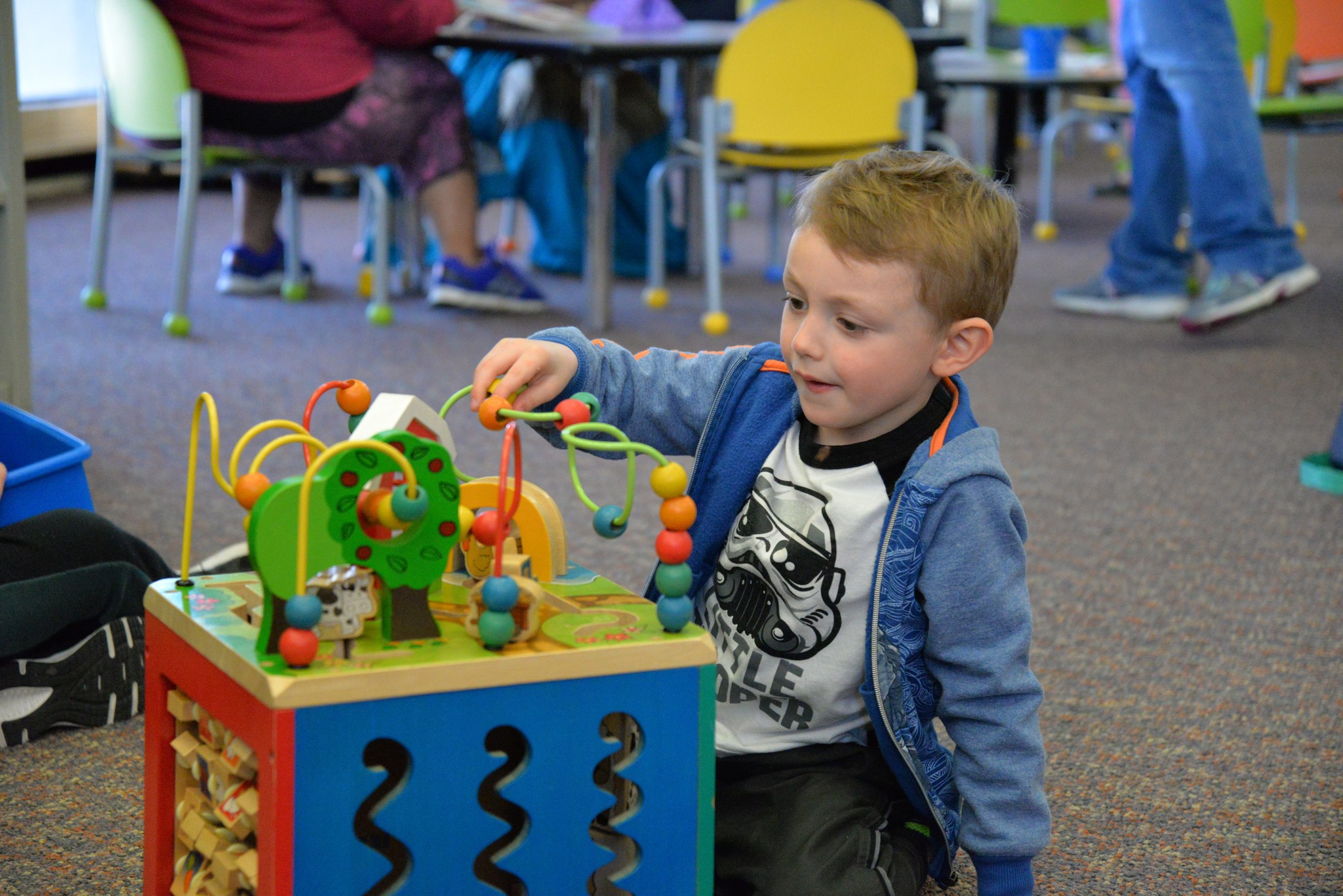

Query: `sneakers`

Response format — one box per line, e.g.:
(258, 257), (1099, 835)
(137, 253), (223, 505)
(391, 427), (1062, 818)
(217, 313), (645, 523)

(427, 244), (548, 315)
(1183, 253), (1321, 331)
(1056, 265), (1192, 320)
(218, 236), (313, 292)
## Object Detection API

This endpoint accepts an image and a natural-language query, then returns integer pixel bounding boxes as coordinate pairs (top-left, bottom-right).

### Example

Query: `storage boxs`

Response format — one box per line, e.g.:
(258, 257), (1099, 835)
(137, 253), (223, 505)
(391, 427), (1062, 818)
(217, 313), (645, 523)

(0, 401), (95, 527)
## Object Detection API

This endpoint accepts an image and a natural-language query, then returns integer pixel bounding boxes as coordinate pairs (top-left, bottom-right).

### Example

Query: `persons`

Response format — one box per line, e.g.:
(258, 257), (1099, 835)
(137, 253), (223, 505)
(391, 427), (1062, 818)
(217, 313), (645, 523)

(1055, 0), (1321, 335)
(470, 146), (1051, 895)
(155, 0), (551, 313)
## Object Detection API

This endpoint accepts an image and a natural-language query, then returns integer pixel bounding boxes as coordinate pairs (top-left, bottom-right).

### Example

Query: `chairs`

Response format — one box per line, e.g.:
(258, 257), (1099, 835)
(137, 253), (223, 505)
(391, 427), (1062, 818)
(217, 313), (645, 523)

(80, 0), (1343, 338)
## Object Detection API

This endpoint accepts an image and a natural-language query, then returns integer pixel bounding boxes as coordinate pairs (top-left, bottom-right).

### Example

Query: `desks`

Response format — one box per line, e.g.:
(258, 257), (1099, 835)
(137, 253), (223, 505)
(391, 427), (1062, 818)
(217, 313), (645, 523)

(401, 19), (972, 337)
